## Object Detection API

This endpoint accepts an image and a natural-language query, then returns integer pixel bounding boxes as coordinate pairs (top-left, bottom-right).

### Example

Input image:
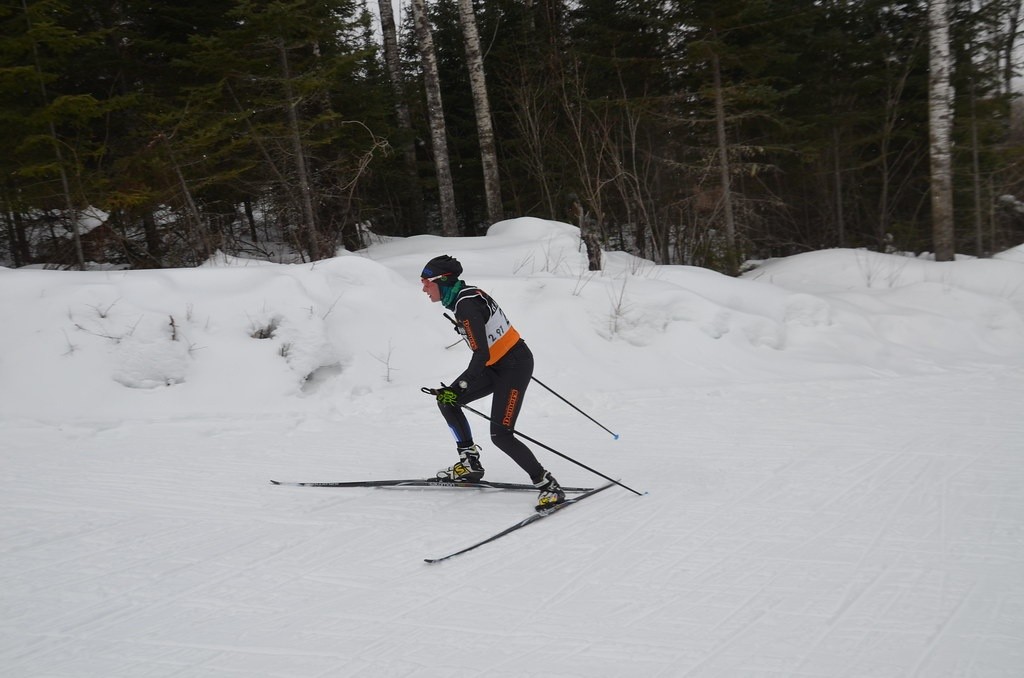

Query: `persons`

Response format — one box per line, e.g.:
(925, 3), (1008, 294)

(421, 255), (565, 512)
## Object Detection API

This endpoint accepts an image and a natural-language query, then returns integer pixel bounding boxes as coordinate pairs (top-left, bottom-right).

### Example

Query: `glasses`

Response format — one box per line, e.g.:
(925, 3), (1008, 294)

(421, 272), (452, 289)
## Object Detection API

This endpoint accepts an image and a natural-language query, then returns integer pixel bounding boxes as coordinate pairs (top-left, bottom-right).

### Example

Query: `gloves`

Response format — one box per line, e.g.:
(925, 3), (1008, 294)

(435, 387), (464, 408)
(454, 319), (463, 335)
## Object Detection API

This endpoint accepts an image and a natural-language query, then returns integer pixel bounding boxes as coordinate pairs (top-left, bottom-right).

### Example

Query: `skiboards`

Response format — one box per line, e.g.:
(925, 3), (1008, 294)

(270, 479), (618, 565)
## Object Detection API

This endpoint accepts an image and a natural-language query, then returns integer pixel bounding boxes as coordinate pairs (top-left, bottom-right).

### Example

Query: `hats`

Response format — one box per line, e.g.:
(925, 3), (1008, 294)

(420, 255), (463, 287)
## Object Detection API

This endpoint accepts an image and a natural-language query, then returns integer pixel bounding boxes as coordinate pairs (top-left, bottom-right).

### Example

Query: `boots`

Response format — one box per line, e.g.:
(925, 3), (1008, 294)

(435, 443), (485, 482)
(532, 471), (566, 512)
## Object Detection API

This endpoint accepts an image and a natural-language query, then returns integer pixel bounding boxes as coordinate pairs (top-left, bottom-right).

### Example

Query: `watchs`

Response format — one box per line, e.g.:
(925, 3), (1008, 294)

(459, 380), (467, 389)
